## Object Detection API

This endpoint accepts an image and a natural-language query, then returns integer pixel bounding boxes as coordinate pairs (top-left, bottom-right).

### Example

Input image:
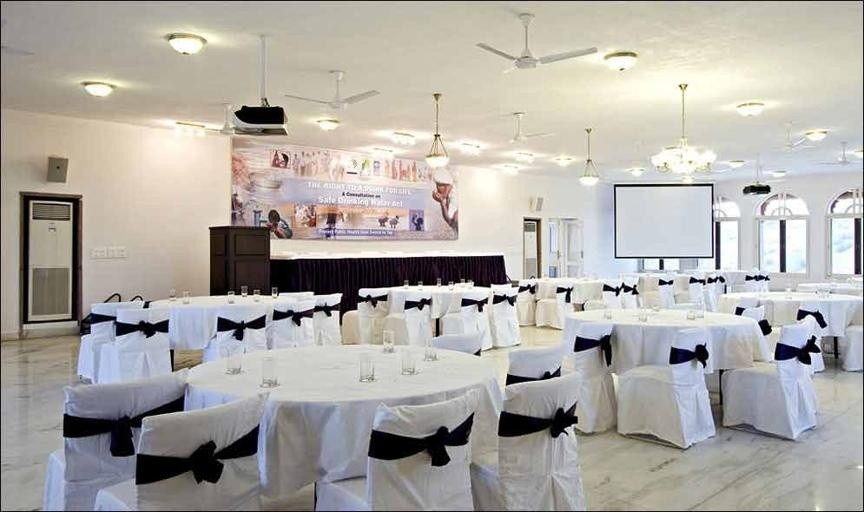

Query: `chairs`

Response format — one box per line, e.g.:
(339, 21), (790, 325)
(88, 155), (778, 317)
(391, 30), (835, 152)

(312, 386), (486, 512)
(616, 324), (712, 449)
(469, 372), (583, 510)
(574, 322), (619, 436)
(94, 392), (272, 510)
(720, 318), (820, 442)
(506, 342), (567, 389)
(516, 267), (864, 373)
(74, 281), (523, 386)
(42, 373), (193, 512)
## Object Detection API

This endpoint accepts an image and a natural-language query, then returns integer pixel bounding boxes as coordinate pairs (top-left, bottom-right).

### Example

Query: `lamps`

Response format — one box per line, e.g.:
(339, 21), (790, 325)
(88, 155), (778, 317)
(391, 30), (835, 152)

(729, 160), (745, 169)
(735, 101), (764, 118)
(649, 83), (717, 174)
(502, 165), (520, 177)
(516, 152), (534, 164)
(628, 168), (646, 177)
(579, 128), (601, 185)
(80, 79), (116, 100)
(802, 128), (827, 142)
(680, 177), (695, 184)
(317, 119), (342, 134)
(602, 48), (640, 73)
(555, 158), (573, 167)
(460, 143), (481, 157)
(388, 133), (417, 147)
(371, 148), (396, 162)
(425, 92), (450, 169)
(164, 30), (205, 57)
(769, 169), (787, 178)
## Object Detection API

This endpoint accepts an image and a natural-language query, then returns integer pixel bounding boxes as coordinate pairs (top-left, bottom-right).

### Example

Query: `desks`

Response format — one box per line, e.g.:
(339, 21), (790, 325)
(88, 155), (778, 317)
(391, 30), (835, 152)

(180, 341), (506, 486)
(272, 252), (509, 311)
(560, 307), (771, 367)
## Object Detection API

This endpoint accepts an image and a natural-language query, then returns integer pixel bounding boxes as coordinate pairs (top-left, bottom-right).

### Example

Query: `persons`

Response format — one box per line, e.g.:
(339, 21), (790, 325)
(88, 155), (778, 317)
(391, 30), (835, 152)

(264, 209), (294, 239)
(269, 149), (430, 182)
(432, 190), (457, 232)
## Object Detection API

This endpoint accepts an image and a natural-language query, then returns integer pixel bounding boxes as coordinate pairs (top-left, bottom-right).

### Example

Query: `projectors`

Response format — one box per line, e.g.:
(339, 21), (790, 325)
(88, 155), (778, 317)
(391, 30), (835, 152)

(743, 185), (771, 194)
(232, 105), (288, 134)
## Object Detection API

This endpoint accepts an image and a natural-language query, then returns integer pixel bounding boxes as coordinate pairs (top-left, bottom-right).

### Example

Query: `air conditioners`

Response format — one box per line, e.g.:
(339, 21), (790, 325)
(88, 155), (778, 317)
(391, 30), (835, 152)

(26, 197), (79, 323)
(524, 219), (538, 280)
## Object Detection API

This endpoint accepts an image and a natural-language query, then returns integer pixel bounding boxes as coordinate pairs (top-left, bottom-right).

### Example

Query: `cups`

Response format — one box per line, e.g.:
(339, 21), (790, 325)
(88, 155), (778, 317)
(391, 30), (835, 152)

(168, 285), (277, 304)
(602, 297), (705, 323)
(402, 277), (475, 291)
(219, 329), (439, 388)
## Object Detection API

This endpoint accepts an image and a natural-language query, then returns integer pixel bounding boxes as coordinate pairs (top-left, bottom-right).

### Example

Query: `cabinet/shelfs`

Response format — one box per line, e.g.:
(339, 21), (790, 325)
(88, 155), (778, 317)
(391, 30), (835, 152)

(205, 224), (272, 298)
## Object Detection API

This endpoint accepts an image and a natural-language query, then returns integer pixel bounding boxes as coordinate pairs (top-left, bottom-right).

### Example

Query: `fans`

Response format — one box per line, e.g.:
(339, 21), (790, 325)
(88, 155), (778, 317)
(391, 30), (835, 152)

(201, 101), (250, 138)
(816, 139), (861, 169)
(473, 11), (599, 75)
(283, 70), (379, 111)
(763, 120), (809, 157)
